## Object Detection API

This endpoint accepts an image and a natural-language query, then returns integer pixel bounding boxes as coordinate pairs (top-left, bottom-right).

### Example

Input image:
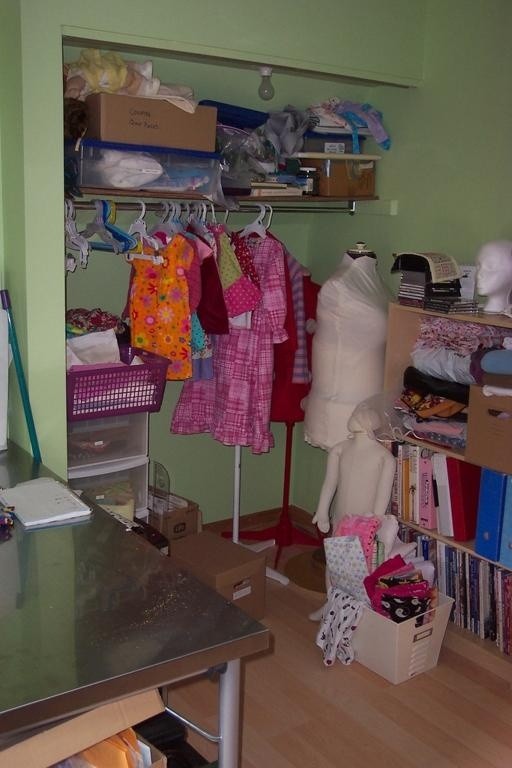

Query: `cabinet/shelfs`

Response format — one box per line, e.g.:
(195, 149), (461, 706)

(66, 409), (149, 528)
(376, 297), (512, 683)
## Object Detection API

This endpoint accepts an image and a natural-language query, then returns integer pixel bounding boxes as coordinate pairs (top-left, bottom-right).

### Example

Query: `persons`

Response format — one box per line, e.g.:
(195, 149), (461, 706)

(475, 238), (512, 312)
(302, 241), (397, 620)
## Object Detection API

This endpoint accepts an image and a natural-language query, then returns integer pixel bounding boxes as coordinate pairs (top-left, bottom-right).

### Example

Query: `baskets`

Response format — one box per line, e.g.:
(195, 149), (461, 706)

(66, 346), (172, 422)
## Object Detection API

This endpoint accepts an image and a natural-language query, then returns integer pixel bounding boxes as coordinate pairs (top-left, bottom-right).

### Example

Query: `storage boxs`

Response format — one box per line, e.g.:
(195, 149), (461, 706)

(464, 381), (512, 475)
(283, 127), (382, 199)
(64, 92), (224, 195)
(145, 484), (268, 623)
(326, 581), (455, 687)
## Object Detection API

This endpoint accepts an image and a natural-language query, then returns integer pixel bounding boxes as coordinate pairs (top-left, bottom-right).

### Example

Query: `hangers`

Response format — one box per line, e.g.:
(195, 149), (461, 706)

(62, 195), (273, 268)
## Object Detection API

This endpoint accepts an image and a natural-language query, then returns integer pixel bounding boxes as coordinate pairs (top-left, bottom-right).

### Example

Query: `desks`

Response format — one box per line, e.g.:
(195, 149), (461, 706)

(0, 436), (270, 768)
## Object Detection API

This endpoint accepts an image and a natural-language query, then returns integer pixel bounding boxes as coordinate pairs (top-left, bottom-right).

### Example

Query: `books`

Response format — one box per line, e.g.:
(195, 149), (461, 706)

(252, 188), (303, 196)
(391, 442), (512, 654)
(0, 476), (93, 528)
